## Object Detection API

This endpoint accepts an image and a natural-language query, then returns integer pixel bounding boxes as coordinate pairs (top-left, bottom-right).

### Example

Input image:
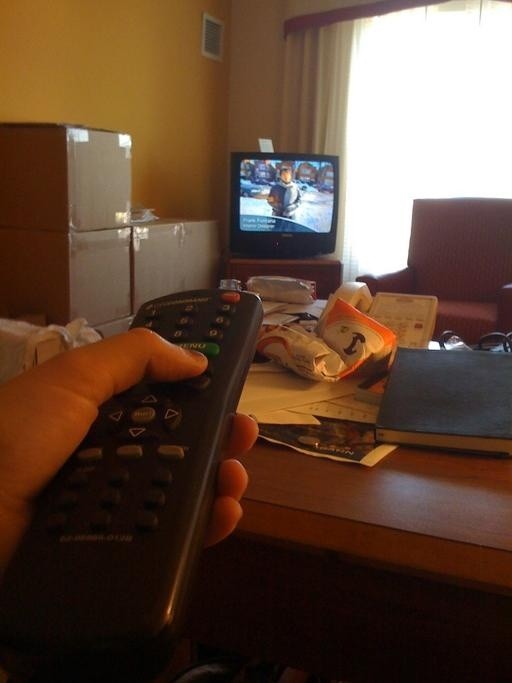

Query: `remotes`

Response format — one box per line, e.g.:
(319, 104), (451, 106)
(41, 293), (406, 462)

(1, 287), (265, 639)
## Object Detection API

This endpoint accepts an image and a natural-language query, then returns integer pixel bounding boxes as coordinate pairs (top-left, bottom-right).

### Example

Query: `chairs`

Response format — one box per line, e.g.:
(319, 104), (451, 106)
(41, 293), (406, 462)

(353, 196), (511, 344)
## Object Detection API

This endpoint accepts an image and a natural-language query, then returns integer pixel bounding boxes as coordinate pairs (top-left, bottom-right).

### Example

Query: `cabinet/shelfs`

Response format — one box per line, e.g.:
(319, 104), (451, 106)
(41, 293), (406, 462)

(221, 257), (344, 303)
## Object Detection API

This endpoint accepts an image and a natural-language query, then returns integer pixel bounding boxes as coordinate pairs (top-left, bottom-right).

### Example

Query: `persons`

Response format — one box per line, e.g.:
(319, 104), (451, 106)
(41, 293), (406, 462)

(1, 324), (262, 683)
(266, 166), (302, 232)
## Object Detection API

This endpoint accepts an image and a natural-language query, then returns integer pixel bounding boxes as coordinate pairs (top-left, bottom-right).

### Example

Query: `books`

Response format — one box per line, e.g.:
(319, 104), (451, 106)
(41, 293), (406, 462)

(373, 344), (511, 459)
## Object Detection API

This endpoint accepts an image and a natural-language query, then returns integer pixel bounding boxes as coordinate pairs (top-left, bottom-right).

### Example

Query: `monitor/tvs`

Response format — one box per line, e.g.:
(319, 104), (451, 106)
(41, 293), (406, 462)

(229, 150), (339, 259)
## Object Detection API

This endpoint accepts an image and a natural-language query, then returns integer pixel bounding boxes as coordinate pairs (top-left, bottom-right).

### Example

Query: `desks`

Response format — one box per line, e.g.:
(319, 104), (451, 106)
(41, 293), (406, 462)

(235, 437), (512, 594)
